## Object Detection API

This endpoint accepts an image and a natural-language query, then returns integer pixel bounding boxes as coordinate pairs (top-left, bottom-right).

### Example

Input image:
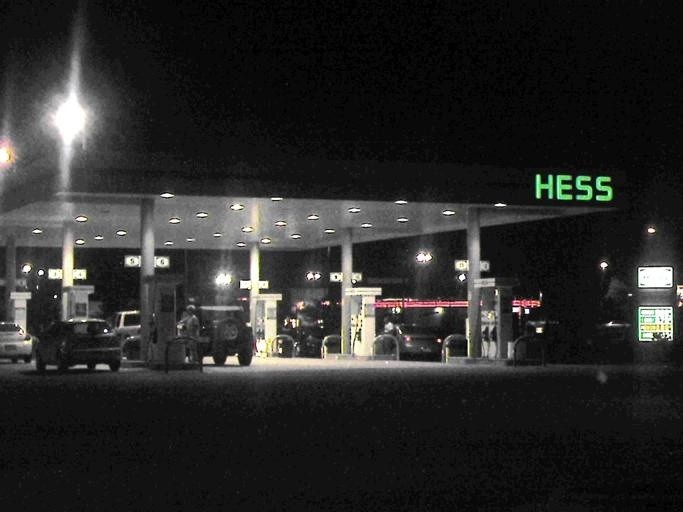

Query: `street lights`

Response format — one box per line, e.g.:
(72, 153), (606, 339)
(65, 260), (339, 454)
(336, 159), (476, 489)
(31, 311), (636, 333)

(306, 271), (321, 281)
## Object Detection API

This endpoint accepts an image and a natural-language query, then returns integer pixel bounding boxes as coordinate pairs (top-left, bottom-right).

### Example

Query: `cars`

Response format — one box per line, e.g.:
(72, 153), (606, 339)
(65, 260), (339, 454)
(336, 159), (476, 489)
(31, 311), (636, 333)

(124, 317), (185, 361)
(598, 320), (631, 334)
(522, 306), (562, 338)
(280, 300), (340, 354)
(373, 321), (442, 361)
(0, 321), (32, 364)
(36, 317), (121, 372)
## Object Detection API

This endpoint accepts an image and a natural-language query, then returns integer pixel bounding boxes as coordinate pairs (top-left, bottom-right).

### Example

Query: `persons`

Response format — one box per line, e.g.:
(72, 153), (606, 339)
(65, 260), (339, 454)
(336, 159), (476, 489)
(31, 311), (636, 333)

(181, 305), (200, 365)
(384, 317), (394, 334)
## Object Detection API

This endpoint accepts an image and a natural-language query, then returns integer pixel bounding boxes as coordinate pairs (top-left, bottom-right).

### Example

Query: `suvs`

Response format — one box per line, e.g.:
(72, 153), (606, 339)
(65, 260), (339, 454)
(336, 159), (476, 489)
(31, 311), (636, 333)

(107, 311), (141, 340)
(178, 305), (253, 365)
